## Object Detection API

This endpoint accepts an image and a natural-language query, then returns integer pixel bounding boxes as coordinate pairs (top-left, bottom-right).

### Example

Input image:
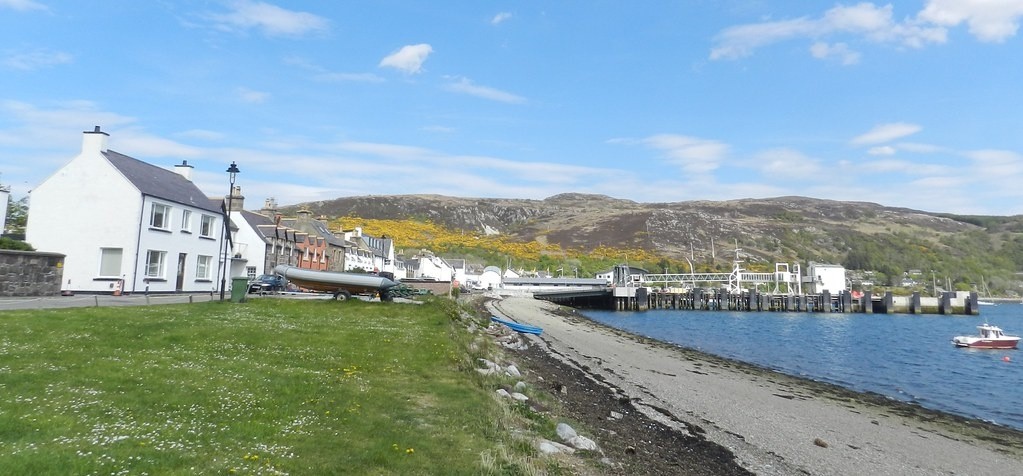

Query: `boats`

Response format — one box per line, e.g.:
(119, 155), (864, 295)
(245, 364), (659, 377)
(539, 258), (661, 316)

(275, 264), (401, 294)
(951, 321), (1021, 349)
(491, 317), (543, 335)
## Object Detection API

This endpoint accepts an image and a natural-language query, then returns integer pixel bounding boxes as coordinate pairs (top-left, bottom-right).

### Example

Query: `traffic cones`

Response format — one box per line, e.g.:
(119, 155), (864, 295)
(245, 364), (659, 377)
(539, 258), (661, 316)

(113, 279), (121, 296)
(61, 280), (74, 296)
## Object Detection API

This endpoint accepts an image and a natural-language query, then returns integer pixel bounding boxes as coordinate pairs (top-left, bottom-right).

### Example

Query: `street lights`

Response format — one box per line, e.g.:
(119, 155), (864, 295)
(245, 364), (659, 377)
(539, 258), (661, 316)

(381, 234), (388, 271)
(219, 161), (241, 301)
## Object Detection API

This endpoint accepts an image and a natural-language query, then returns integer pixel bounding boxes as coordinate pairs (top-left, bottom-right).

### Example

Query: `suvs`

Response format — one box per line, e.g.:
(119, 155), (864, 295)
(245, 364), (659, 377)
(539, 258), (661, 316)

(248, 275), (289, 294)
